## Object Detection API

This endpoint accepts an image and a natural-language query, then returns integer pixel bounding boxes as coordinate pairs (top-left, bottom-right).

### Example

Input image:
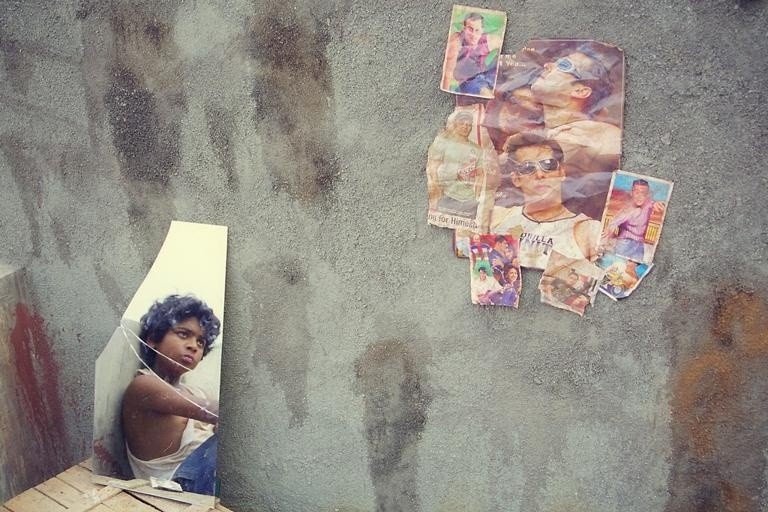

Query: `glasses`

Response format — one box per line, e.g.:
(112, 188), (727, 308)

(504, 92), (541, 119)
(514, 159), (560, 176)
(555, 57), (581, 79)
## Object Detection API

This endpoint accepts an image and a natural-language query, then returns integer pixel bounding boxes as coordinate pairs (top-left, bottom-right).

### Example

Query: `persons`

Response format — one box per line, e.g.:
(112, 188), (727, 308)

(529, 50), (619, 172)
(603, 258), (640, 291)
(483, 64), (544, 155)
(603, 179), (668, 259)
(428, 111), (487, 219)
(468, 234), (520, 306)
(547, 272), (580, 293)
(118, 293), (222, 498)
(441, 12), (498, 99)
(489, 131), (603, 270)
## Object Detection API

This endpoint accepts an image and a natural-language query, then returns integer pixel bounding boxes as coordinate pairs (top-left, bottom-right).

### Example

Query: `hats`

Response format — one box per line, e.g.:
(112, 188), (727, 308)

(454, 111), (472, 121)
